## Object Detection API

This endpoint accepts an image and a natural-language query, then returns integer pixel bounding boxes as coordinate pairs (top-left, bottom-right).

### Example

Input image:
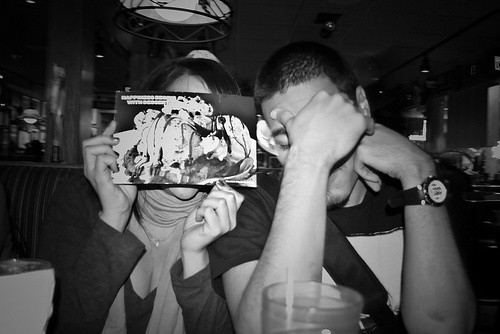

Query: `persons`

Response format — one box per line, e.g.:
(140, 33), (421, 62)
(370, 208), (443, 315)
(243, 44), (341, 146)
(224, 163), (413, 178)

(47, 38), (477, 334)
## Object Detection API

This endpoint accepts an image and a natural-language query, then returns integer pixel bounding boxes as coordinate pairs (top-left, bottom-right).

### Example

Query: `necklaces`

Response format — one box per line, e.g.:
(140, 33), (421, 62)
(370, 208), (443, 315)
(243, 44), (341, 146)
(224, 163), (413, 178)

(139, 218), (177, 247)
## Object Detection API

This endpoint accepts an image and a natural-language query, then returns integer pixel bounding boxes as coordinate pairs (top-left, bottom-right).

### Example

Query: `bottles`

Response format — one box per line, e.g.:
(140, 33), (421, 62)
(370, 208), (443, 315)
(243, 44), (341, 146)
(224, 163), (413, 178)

(52, 138), (61, 163)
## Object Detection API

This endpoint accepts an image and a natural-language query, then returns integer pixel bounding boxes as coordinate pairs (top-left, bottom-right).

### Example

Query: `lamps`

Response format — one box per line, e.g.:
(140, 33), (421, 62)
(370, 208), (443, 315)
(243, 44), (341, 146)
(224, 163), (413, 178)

(113, 0), (232, 43)
(314, 12), (344, 31)
(419, 62), (430, 73)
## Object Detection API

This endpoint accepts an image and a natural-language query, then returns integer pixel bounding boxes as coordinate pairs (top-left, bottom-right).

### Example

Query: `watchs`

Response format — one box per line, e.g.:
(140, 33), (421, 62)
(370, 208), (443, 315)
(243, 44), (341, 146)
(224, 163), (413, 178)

(388, 175), (448, 208)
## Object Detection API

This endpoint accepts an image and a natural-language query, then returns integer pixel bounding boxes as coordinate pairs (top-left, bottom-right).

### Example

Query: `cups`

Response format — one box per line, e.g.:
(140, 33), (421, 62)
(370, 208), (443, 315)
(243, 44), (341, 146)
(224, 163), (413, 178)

(261, 280), (363, 334)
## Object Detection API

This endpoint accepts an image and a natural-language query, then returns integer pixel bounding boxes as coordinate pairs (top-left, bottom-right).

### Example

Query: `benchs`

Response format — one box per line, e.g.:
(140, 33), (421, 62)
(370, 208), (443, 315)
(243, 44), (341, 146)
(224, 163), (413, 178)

(0, 160), (83, 259)
